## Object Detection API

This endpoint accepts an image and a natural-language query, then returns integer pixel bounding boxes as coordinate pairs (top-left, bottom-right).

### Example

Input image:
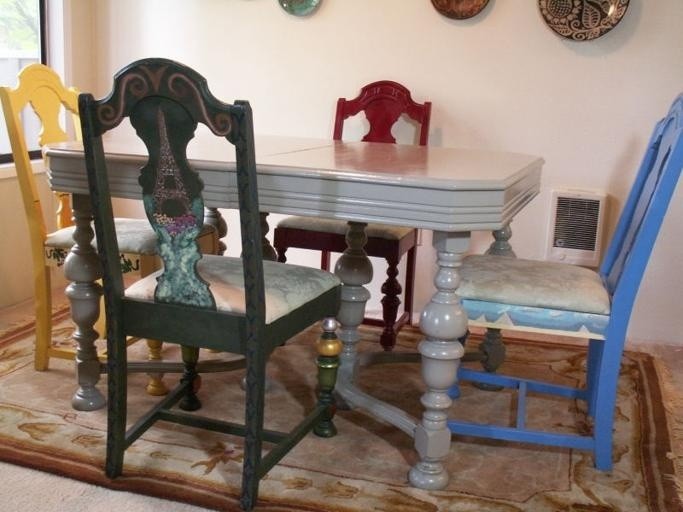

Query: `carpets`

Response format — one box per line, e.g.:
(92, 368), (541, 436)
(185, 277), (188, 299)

(0, 307), (683, 512)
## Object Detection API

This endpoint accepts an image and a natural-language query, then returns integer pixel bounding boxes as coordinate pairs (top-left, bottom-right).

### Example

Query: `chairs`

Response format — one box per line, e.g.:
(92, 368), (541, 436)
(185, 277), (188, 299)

(448, 93), (683, 472)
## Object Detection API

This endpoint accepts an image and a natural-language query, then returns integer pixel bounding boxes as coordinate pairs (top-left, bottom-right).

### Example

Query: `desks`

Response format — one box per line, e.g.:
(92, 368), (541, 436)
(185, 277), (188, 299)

(41, 127), (545, 491)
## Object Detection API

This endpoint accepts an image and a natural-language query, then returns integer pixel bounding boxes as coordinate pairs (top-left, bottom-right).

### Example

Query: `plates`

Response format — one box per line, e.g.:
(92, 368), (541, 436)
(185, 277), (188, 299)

(538, 0), (630, 42)
(429, 0), (490, 21)
(277, 0), (320, 18)
